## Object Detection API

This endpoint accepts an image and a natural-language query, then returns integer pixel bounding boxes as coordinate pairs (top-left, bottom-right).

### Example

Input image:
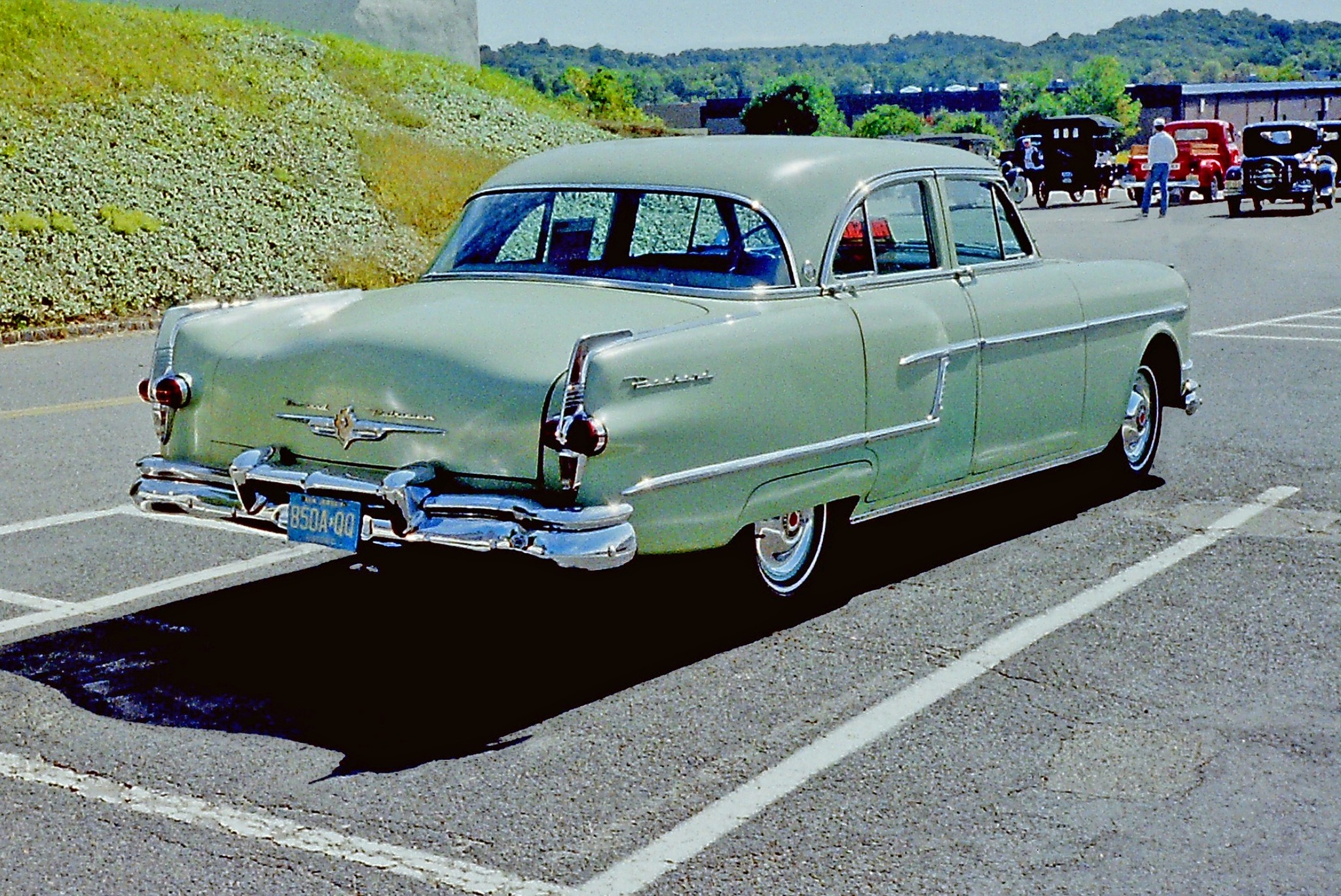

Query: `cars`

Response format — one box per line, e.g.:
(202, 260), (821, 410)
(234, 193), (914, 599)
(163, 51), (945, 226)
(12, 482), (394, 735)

(1034, 116), (1127, 209)
(1222, 120), (1341, 216)
(120, 131), (1208, 615)
(914, 133), (1028, 205)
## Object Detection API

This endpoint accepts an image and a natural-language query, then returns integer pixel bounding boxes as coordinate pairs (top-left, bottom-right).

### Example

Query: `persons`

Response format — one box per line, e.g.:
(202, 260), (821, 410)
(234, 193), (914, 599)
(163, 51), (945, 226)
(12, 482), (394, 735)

(1142, 119), (1178, 218)
(1021, 137), (1044, 172)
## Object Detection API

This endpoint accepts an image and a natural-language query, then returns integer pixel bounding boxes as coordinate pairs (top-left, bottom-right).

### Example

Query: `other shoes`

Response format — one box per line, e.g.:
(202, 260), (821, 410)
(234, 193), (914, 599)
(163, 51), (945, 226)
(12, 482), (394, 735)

(1141, 211), (1149, 216)
(1159, 212), (1166, 217)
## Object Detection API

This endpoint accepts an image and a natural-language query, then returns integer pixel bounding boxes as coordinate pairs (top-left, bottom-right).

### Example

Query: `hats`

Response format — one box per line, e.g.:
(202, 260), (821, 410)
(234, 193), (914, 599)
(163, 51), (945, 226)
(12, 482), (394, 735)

(1153, 118), (1167, 127)
(1022, 138), (1031, 142)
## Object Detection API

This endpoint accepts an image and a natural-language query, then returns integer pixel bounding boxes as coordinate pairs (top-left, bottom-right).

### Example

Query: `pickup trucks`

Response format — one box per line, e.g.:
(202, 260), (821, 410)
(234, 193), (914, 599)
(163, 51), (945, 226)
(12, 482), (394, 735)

(1119, 118), (1242, 205)
